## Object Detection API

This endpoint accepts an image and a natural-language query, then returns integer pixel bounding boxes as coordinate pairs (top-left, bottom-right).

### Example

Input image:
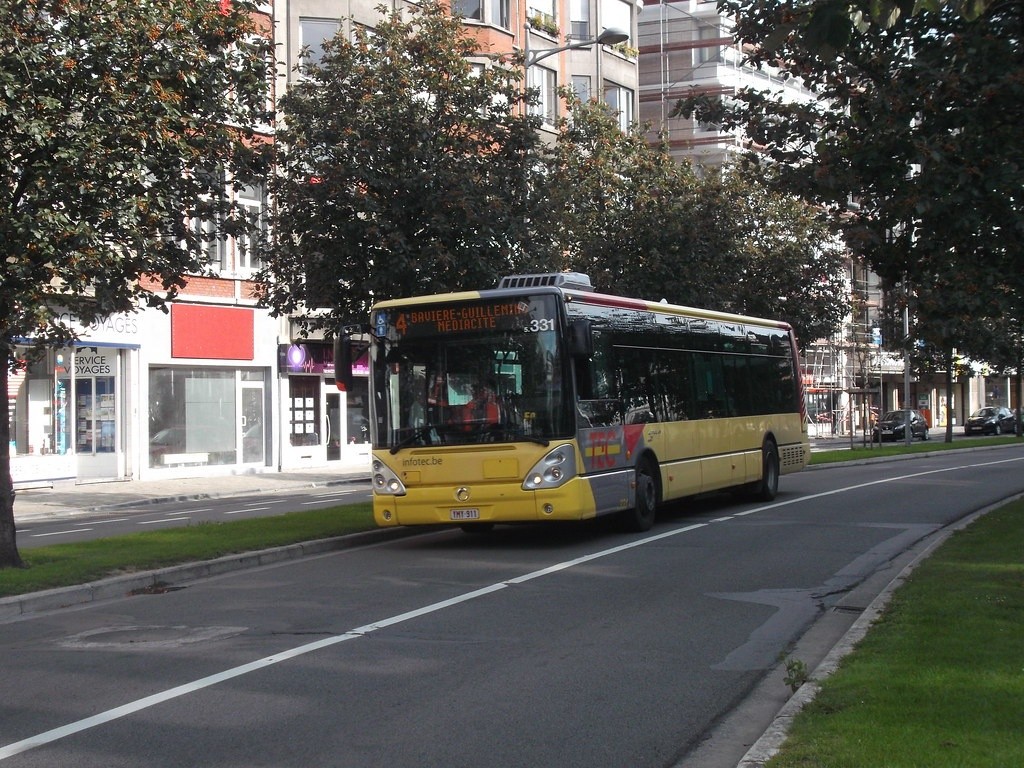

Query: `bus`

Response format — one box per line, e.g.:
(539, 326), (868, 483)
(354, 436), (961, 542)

(332, 270), (810, 535)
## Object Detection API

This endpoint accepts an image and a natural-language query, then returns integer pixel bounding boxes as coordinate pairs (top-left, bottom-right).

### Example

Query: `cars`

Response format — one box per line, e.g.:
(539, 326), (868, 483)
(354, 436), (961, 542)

(871, 409), (931, 442)
(1012, 406), (1024, 439)
(965, 405), (1015, 436)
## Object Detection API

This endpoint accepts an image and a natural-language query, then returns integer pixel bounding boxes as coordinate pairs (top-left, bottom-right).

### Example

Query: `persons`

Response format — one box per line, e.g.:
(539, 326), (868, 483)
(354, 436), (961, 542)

(463, 383), (497, 433)
